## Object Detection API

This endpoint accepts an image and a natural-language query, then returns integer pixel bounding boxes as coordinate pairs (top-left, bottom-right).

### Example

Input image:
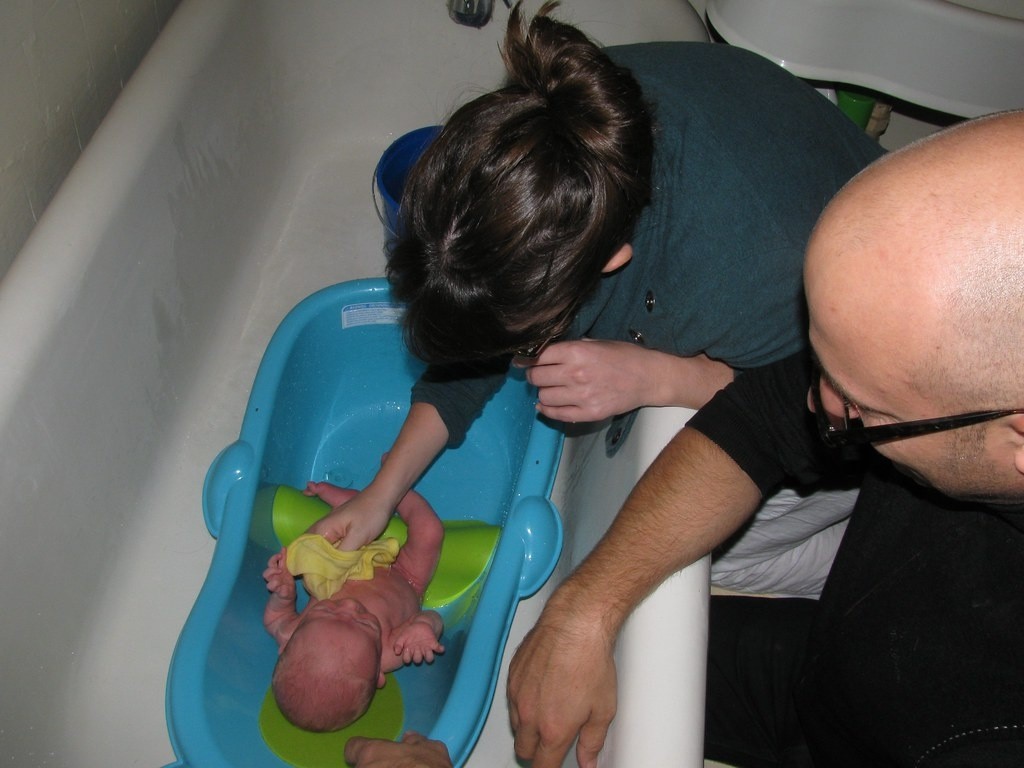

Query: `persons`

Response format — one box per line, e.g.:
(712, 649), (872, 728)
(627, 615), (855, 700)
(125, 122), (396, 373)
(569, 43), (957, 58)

(307, 0), (896, 556)
(260, 454), (444, 735)
(506, 111), (1020, 767)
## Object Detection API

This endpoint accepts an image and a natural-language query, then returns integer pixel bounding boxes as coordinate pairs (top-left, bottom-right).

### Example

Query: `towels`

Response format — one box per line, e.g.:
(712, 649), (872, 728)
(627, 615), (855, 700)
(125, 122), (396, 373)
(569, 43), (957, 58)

(286, 533), (400, 601)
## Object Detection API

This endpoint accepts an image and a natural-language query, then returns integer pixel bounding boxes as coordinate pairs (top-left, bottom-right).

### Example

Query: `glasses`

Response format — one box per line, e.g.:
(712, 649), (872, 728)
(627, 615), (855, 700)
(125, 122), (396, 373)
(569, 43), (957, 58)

(800, 356), (1024, 452)
(519, 272), (608, 360)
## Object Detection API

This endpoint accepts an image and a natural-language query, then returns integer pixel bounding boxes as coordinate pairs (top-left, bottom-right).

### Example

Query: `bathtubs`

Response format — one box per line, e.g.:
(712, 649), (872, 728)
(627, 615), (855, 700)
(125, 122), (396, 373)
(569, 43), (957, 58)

(0, 1), (710, 768)
